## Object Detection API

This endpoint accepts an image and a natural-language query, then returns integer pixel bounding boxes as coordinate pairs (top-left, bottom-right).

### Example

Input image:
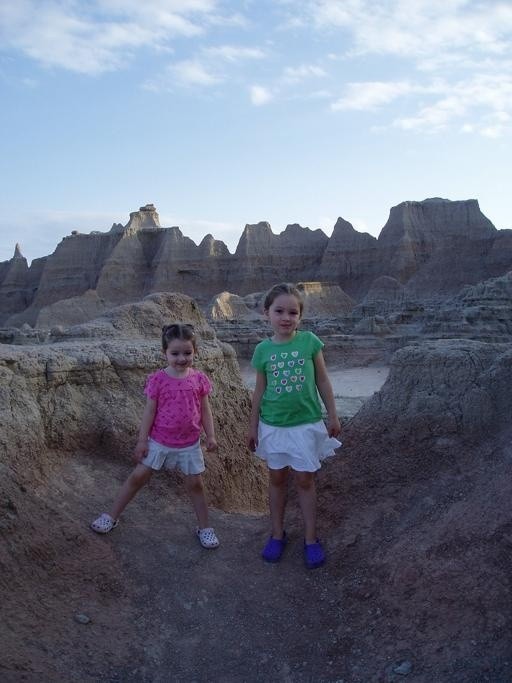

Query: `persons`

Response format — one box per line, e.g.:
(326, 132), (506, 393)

(245, 281), (345, 569)
(89, 320), (222, 550)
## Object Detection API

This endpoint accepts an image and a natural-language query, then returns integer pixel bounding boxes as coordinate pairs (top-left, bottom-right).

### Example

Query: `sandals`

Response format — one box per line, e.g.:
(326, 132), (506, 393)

(304, 537), (323, 569)
(197, 527), (219, 549)
(262, 530), (286, 563)
(91, 513), (119, 533)
